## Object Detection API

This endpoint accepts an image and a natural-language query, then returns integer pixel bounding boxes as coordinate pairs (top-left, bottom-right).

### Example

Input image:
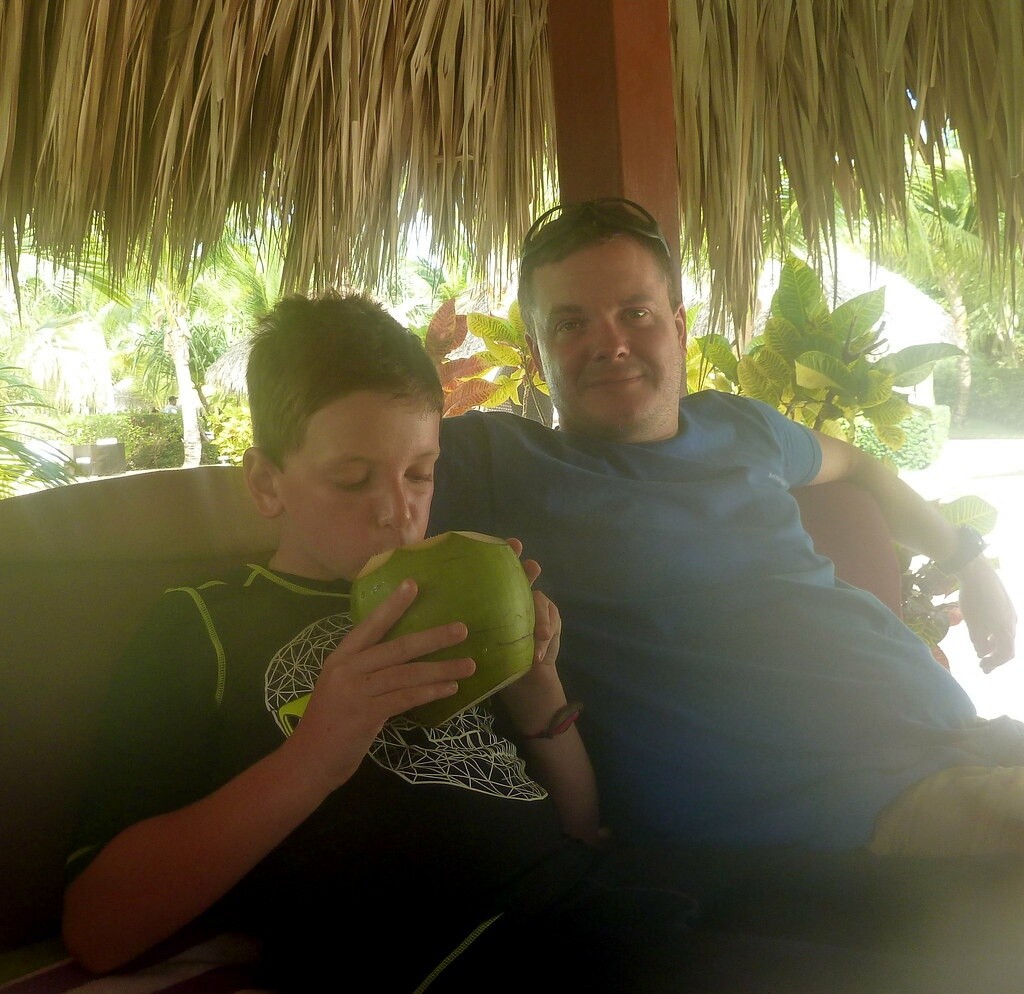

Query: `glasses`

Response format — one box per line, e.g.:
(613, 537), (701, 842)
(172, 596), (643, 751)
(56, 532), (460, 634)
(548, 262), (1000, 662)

(518, 198), (670, 285)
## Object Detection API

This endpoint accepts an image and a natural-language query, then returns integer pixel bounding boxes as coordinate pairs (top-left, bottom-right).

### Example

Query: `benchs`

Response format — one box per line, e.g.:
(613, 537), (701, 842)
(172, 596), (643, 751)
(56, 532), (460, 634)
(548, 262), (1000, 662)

(0, 469), (949, 994)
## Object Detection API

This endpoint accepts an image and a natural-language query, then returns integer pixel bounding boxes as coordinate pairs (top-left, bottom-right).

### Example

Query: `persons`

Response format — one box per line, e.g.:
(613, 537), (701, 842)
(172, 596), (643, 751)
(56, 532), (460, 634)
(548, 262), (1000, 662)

(60, 291), (932, 994)
(1, 198), (1024, 994)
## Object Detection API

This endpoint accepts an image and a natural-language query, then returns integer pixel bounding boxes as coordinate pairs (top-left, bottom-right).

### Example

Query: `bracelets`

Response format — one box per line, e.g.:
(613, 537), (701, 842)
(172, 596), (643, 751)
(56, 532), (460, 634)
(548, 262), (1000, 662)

(513, 698), (581, 743)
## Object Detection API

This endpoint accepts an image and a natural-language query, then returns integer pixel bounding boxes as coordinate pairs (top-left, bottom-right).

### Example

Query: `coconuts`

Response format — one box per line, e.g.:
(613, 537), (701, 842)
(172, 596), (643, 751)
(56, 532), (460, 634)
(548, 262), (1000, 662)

(350, 531), (534, 731)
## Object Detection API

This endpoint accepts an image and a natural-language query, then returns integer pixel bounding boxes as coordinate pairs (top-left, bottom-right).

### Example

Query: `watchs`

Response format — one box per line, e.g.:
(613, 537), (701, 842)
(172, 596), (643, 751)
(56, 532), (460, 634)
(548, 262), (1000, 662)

(942, 522), (986, 575)
